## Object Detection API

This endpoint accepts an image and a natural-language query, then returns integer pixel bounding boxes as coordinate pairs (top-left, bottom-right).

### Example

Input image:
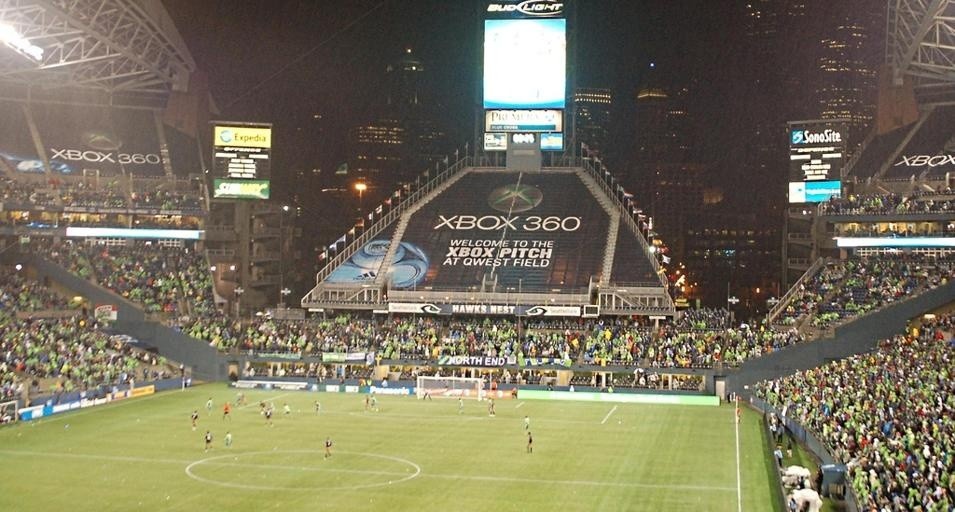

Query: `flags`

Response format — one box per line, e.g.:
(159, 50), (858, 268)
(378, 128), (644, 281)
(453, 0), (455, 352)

(313, 139), (468, 262)
(582, 141), (671, 266)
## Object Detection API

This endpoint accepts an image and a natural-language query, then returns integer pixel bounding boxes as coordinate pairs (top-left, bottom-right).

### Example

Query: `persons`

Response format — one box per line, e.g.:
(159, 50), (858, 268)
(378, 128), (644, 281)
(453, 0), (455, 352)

(202, 429), (213, 452)
(723, 193), (955, 512)
(225, 308), (722, 425)
(189, 409), (198, 432)
(205, 395), (213, 418)
(224, 430), (232, 448)
(523, 415), (530, 430)
(1, 177), (223, 410)
(324, 436), (333, 458)
(526, 431), (533, 453)
